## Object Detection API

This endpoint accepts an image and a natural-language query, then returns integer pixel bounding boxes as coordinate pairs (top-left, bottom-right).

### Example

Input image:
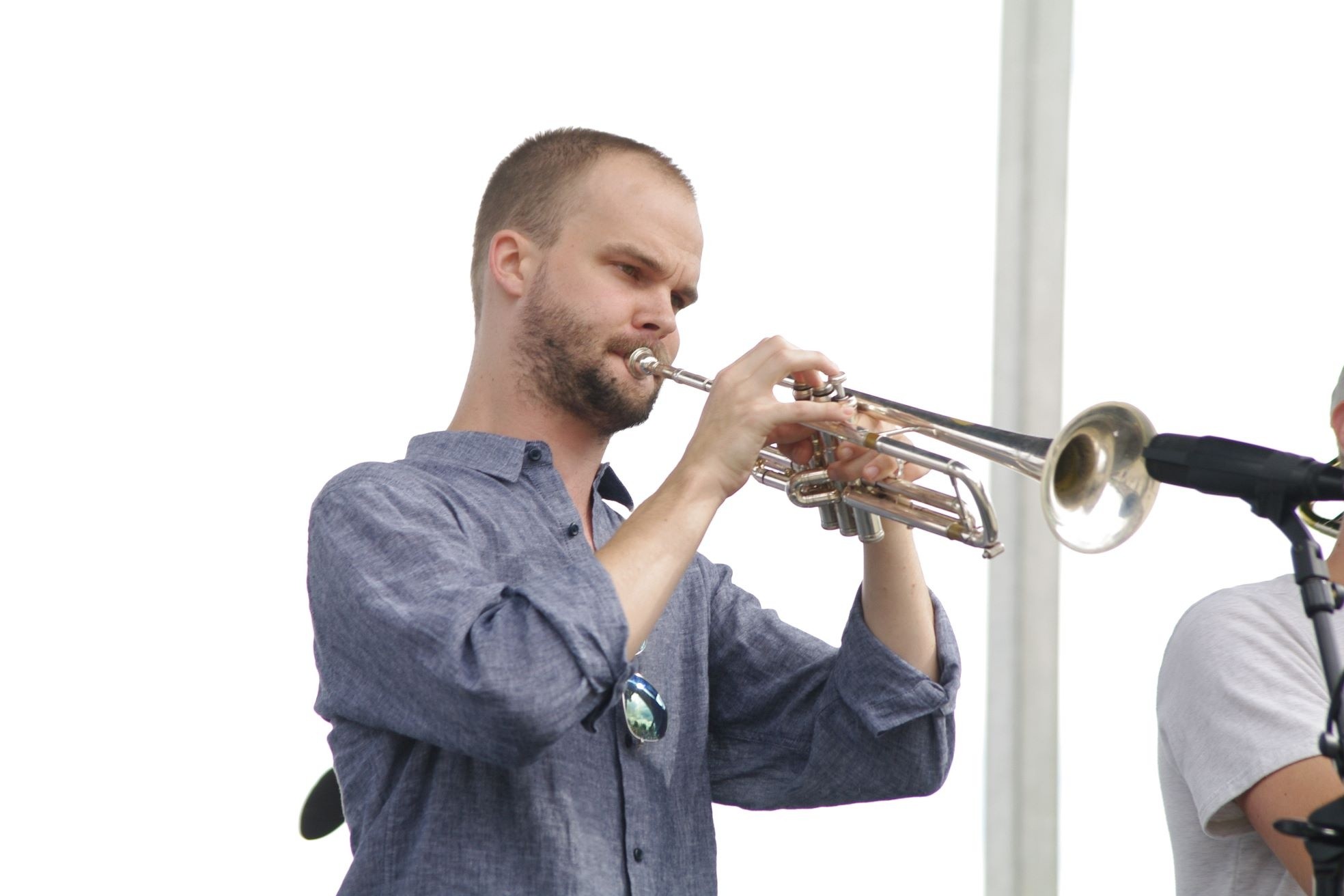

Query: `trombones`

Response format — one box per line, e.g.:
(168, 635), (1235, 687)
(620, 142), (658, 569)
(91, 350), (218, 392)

(1300, 455), (1343, 538)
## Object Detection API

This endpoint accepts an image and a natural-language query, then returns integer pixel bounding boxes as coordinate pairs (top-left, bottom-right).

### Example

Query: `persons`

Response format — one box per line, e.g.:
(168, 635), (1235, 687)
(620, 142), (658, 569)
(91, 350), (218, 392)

(1154, 364), (1344, 896)
(306, 125), (962, 896)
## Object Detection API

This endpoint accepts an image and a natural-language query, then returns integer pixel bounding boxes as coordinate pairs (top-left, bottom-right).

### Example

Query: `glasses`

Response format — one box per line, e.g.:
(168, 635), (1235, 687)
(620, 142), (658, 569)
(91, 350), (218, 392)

(620, 639), (668, 748)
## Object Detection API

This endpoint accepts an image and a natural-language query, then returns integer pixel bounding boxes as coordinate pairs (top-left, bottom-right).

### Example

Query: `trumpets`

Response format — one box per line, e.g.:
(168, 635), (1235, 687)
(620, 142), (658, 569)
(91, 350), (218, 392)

(627, 347), (1157, 559)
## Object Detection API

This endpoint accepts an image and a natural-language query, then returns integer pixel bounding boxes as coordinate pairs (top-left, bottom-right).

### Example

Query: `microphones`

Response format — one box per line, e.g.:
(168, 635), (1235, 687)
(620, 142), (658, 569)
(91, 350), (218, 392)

(299, 765), (344, 841)
(1141, 431), (1343, 506)
